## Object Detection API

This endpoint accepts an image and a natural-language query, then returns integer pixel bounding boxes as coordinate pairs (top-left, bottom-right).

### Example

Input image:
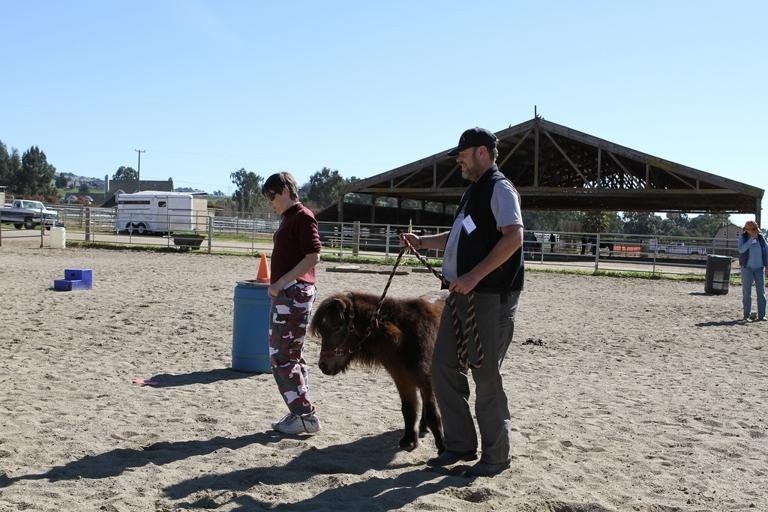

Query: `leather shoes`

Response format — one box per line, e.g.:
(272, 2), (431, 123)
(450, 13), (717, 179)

(427, 450), (478, 466)
(466, 456), (512, 478)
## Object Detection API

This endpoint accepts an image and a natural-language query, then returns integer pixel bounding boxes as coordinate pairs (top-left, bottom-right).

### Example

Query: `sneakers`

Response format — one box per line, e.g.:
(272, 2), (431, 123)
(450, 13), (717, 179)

(276, 415), (320, 435)
(271, 414), (291, 432)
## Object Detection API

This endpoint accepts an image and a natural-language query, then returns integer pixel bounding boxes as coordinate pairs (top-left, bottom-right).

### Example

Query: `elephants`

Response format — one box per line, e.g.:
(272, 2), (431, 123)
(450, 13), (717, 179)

(580, 230), (614, 256)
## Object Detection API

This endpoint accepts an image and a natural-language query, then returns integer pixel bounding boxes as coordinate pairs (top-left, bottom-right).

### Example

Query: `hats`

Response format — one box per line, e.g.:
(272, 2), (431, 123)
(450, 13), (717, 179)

(446, 127), (497, 156)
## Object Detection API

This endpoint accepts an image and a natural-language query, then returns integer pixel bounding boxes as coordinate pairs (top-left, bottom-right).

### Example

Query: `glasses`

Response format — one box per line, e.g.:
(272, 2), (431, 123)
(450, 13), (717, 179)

(266, 191), (276, 201)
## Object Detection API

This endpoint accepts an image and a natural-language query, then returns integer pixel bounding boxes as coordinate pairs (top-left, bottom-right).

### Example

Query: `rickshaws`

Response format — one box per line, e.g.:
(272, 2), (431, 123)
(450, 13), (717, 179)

(163, 235), (207, 251)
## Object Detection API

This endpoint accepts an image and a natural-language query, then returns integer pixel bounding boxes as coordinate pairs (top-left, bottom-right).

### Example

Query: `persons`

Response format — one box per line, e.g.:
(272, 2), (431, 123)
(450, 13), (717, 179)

(399, 125), (525, 477)
(737, 221), (768, 324)
(581, 235), (587, 255)
(261, 171), (322, 435)
(548, 233), (555, 253)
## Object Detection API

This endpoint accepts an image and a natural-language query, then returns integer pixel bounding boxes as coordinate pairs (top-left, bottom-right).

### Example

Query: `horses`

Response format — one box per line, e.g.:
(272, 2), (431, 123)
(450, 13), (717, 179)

(307, 290), (514, 456)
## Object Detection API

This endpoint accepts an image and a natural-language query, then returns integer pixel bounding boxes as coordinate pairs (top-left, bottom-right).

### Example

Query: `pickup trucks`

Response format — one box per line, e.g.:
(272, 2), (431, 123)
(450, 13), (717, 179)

(0, 200), (57, 231)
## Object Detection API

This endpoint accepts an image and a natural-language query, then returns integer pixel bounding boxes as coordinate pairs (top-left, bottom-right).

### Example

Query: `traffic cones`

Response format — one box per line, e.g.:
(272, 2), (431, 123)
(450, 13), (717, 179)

(256, 254), (272, 283)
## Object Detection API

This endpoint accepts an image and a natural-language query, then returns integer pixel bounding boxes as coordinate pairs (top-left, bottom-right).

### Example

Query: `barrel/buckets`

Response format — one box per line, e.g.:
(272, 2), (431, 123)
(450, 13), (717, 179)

(232, 277), (272, 374)
